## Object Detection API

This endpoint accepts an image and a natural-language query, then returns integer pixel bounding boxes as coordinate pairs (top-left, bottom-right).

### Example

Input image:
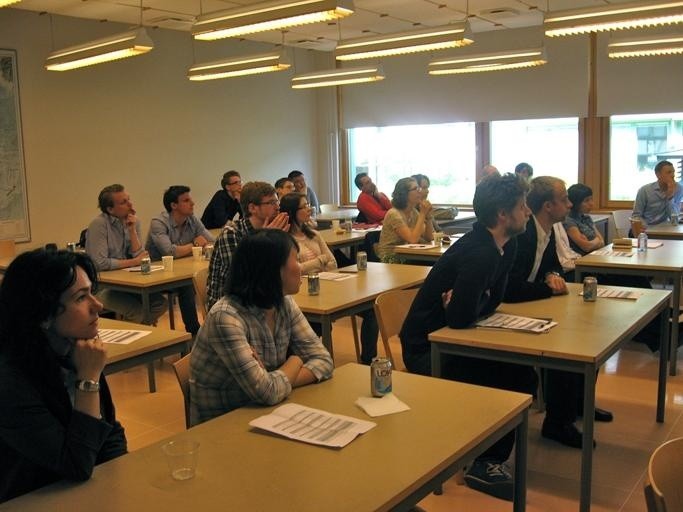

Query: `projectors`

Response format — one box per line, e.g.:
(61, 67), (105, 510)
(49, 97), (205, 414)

(462, 4), (547, 50)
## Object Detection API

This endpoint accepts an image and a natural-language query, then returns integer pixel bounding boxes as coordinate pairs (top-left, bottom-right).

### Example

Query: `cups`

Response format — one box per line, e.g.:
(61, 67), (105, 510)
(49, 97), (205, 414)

(162, 256), (174, 270)
(191, 247), (203, 261)
(192, 256), (204, 270)
(331, 220), (339, 230)
(159, 439), (200, 481)
(433, 233), (443, 245)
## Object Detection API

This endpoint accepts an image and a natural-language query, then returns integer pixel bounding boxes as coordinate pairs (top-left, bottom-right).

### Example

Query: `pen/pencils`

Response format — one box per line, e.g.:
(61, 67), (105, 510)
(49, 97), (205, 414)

(410, 245), (425, 247)
(338, 271), (359, 273)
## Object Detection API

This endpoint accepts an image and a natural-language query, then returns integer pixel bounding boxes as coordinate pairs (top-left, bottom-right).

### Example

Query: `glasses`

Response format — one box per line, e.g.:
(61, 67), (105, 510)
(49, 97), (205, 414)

(229, 181), (241, 185)
(261, 200), (280, 206)
(298, 202), (310, 210)
(408, 186), (422, 193)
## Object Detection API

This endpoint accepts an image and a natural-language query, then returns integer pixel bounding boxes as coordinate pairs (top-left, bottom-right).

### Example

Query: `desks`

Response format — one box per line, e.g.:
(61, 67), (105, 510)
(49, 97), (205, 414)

(316, 208), (360, 222)
(317, 224), (384, 263)
(95, 254), (213, 326)
(573, 236), (683, 377)
(207, 228), (224, 241)
(644, 218), (683, 238)
(427, 282), (674, 512)
(95, 316), (194, 431)
(391, 233), (469, 266)
(435, 210), (477, 230)
(585, 213), (614, 246)
(293, 260), (434, 368)
(1, 360), (535, 512)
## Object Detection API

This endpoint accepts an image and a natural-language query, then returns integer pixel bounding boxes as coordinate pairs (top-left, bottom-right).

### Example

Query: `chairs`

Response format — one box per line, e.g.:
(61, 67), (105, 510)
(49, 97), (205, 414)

(642, 436), (683, 512)
(172, 351), (191, 429)
(372, 288), (422, 372)
(193, 266), (209, 320)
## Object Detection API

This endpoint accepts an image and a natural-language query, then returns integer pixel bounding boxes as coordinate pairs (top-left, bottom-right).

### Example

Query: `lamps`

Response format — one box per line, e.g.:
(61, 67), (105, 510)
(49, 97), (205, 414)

(334, 1), (474, 63)
(428, 20), (548, 76)
(290, 26), (385, 90)
(186, 30), (292, 82)
(188, 0), (356, 42)
(607, 33), (683, 60)
(41, 0), (154, 73)
(542, 1), (683, 39)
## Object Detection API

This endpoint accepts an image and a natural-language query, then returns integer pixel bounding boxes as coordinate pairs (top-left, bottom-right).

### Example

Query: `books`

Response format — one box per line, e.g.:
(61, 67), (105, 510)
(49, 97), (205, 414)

(632, 240), (664, 249)
(611, 238), (633, 252)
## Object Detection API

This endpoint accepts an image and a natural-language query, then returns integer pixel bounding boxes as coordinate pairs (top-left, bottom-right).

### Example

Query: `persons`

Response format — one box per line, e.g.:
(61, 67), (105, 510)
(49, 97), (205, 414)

(142, 185), (215, 344)
(84, 183), (169, 325)
(378, 177), (434, 264)
(188, 229), (335, 428)
(280, 193), (379, 365)
(479, 165), (501, 181)
(561, 184), (683, 322)
(201, 170), (244, 230)
(0, 248), (128, 503)
(554, 223), (683, 353)
(515, 163), (534, 181)
(628, 160), (683, 285)
(288, 171), (353, 268)
(355, 172), (393, 258)
(503, 176), (613, 451)
(275, 177), (295, 205)
(205, 181), (291, 311)
(411, 174), (445, 243)
(398, 170), (533, 501)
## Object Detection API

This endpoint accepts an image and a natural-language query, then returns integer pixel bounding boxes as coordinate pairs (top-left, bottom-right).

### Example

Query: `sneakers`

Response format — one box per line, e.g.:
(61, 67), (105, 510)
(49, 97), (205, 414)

(464, 462), (513, 502)
(541, 420), (596, 451)
(594, 407), (613, 422)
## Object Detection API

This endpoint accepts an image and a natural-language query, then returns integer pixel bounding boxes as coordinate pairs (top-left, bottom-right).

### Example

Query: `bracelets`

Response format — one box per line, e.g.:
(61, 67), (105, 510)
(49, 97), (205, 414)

(317, 256), (325, 266)
(425, 215), (434, 220)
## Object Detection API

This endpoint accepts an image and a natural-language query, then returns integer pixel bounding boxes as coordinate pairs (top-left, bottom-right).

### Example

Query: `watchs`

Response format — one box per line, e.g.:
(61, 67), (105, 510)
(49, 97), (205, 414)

(190, 240), (200, 247)
(546, 271), (560, 276)
(73, 379), (100, 393)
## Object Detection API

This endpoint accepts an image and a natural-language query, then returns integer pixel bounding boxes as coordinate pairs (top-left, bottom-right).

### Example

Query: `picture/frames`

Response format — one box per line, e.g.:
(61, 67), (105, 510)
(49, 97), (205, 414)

(1, 46), (32, 246)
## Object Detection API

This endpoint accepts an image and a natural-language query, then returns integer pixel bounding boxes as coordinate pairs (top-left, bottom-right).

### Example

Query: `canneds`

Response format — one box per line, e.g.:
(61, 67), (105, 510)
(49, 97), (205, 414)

(583, 276), (598, 303)
(141, 258), (151, 274)
(371, 357), (393, 397)
(204, 244), (214, 259)
(357, 252), (367, 270)
(307, 272), (320, 296)
(345, 221), (353, 233)
(637, 231), (647, 252)
